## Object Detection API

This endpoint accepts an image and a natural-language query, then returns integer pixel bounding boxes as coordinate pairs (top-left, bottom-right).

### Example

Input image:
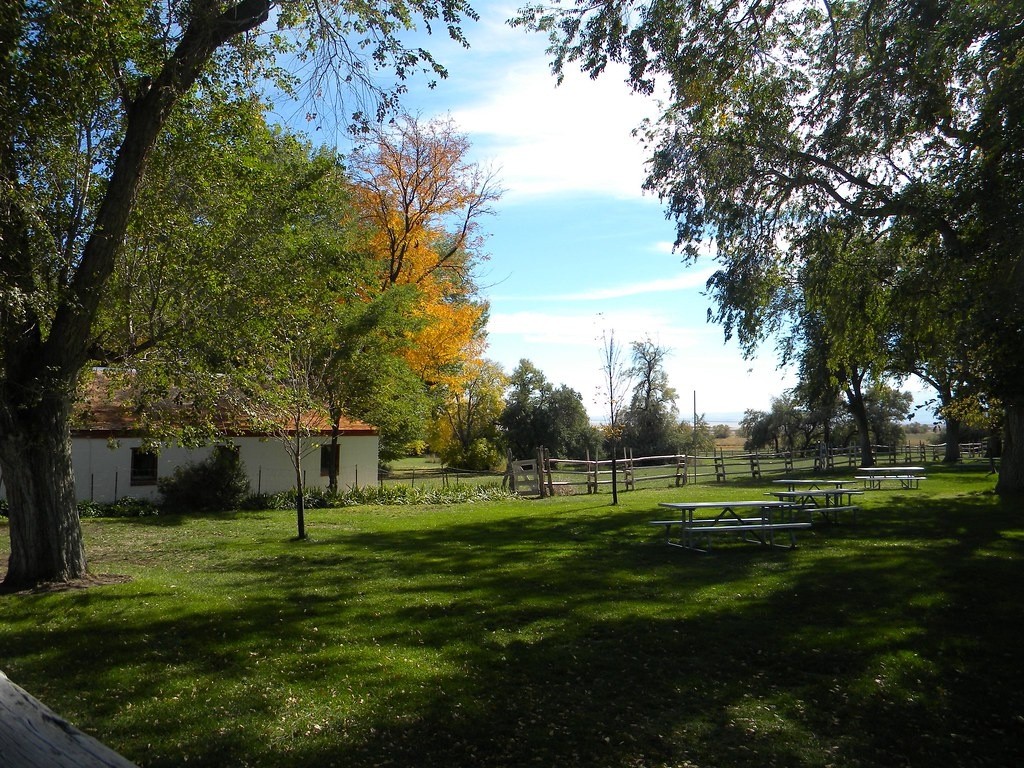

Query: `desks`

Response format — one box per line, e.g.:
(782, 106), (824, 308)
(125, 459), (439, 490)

(771, 489), (860, 526)
(858, 467), (925, 489)
(657, 501), (796, 553)
(773, 479), (859, 512)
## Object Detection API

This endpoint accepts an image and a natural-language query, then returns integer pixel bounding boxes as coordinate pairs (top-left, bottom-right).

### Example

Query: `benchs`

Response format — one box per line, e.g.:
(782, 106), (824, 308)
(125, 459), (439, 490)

(647, 475), (927, 532)
